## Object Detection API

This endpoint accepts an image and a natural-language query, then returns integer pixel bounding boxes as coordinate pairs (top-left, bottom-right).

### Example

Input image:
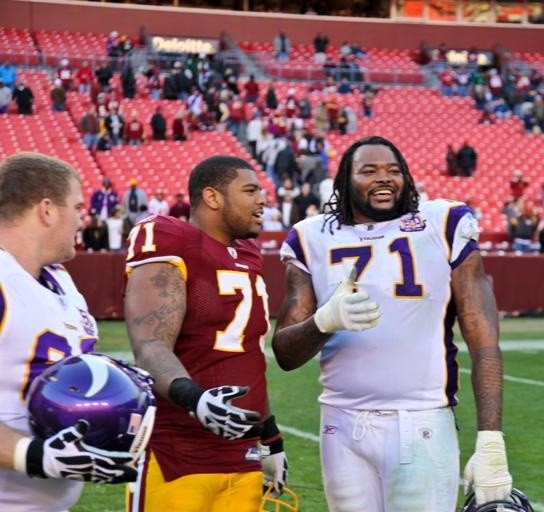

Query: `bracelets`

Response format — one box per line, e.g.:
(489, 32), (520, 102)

(169, 377), (199, 407)
(259, 416), (283, 455)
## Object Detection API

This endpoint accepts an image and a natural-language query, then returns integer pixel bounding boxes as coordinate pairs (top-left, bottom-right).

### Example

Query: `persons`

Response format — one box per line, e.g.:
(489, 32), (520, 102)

(124, 156), (287, 512)
(418, 40), (543, 136)
(415, 169), (543, 254)
(0, 31), (382, 254)
(271, 136), (512, 512)
(446, 140), (477, 177)
(0, 152), (139, 512)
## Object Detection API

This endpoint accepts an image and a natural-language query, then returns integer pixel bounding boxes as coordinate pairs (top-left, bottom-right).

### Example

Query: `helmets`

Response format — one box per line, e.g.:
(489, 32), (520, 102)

(460, 484), (534, 512)
(23, 349), (160, 465)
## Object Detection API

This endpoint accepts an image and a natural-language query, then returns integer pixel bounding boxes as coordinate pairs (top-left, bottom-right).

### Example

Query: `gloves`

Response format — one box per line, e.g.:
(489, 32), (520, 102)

(461, 429), (514, 508)
(10, 417), (139, 485)
(310, 262), (383, 342)
(254, 414), (289, 499)
(169, 376), (263, 441)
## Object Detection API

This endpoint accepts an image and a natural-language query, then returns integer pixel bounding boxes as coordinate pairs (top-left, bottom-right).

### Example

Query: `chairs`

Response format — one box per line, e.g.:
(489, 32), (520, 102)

(1, 20), (542, 254)
